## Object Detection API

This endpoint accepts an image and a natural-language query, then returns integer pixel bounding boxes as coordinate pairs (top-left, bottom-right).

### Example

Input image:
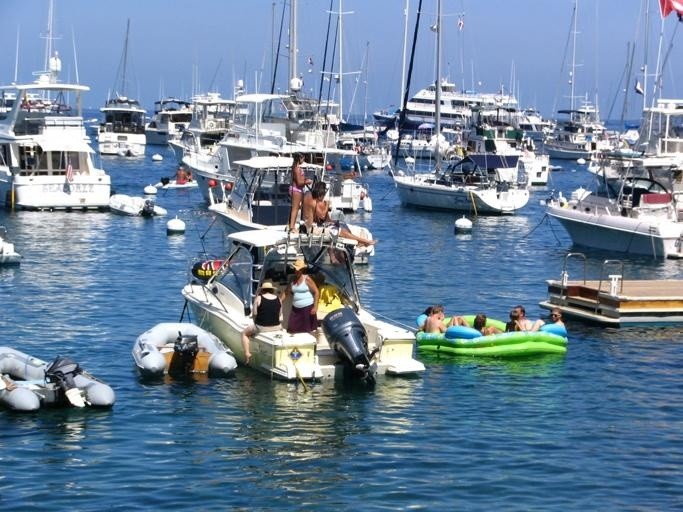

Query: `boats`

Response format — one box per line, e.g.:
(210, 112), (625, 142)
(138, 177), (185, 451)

(108, 193), (168, 217)
(0, 224), (23, 263)
(180, 223), (426, 391)
(131, 321), (238, 379)
(0, 346), (115, 413)
(415, 314), (569, 357)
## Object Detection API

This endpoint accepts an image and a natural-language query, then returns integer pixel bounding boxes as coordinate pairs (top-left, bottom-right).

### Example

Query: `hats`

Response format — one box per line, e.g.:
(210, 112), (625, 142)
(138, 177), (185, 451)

(293, 260), (307, 271)
(259, 283), (277, 291)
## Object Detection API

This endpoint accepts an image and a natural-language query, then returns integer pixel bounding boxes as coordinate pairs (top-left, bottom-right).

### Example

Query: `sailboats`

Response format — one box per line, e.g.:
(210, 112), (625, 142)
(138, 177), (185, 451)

(1, 0), (111, 211)
(96, 18), (147, 158)
(544, 26), (683, 260)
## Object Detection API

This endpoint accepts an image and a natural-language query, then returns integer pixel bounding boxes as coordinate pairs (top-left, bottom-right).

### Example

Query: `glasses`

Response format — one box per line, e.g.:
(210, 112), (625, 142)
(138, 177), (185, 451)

(551, 314), (558, 317)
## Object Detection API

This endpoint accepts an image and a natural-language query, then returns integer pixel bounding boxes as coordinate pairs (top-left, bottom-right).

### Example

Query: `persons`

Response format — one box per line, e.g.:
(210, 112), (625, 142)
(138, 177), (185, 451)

(21, 99), (30, 108)
(172, 167), (193, 185)
(302, 182), (341, 264)
(313, 187), (379, 247)
(311, 272), (349, 326)
(353, 143), (360, 155)
(289, 152), (312, 233)
(418, 305), (567, 336)
(241, 282), (283, 366)
(280, 262), (319, 340)
